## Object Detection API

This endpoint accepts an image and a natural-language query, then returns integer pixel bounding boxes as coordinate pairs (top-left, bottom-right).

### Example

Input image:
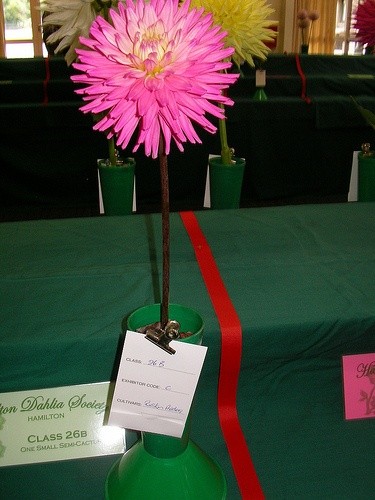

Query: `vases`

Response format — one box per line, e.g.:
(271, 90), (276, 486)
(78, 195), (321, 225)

(94, 157), (138, 215)
(98, 301), (229, 500)
(300, 43), (309, 55)
(205, 153), (247, 209)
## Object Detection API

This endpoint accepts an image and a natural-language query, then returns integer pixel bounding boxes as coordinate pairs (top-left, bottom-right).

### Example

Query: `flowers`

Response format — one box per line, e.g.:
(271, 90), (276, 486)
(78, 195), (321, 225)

(347, 0), (375, 51)
(66, 0), (241, 334)
(33, 0), (130, 68)
(296, 9), (319, 46)
(178, 0), (284, 162)
